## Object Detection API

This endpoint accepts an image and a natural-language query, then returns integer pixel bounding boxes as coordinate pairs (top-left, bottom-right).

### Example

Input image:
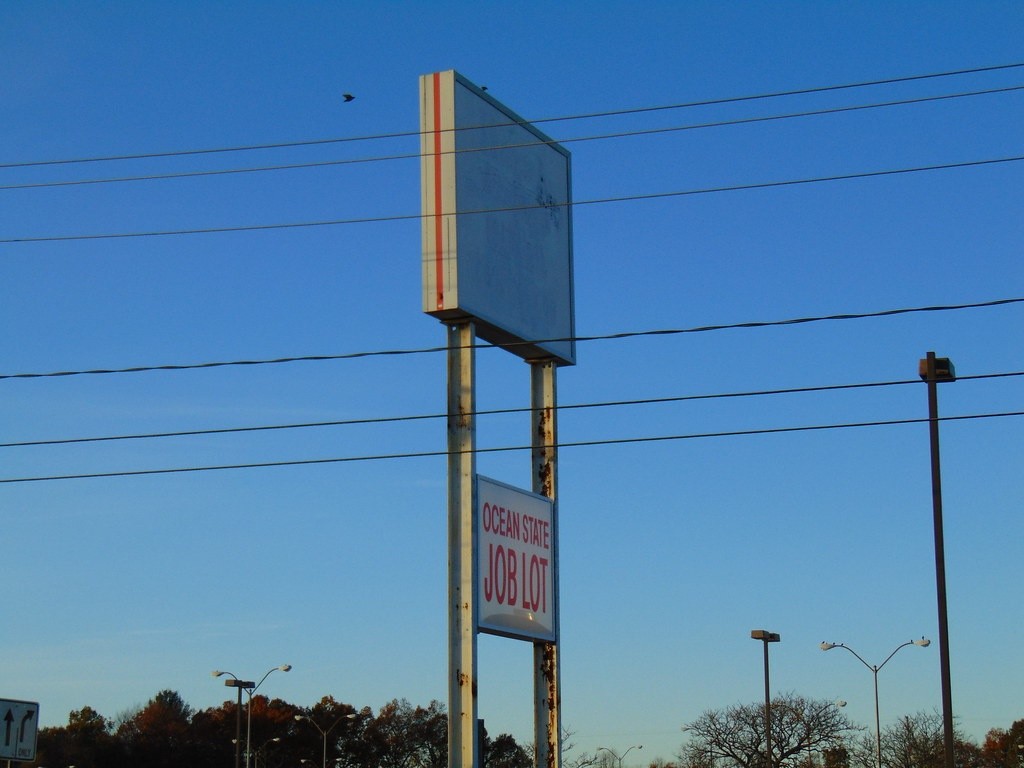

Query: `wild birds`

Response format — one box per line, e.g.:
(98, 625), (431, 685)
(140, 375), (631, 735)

(481, 86), (488, 93)
(343, 94), (356, 103)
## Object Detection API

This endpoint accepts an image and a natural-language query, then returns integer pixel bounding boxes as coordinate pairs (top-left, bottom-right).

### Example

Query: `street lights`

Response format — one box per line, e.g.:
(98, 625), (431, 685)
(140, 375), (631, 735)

(596, 742), (644, 768)
(210, 660), (292, 767)
(820, 634), (931, 768)
